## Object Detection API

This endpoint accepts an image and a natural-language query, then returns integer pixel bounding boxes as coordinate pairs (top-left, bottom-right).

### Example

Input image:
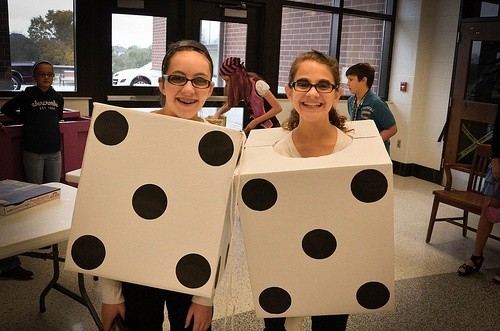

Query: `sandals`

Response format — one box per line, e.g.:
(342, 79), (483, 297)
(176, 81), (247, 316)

(458, 253), (484, 276)
(493, 273), (500, 283)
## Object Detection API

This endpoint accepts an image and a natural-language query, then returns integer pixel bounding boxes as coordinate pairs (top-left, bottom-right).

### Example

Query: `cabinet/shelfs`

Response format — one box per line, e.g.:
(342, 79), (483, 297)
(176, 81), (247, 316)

(441, 21), (500, 168)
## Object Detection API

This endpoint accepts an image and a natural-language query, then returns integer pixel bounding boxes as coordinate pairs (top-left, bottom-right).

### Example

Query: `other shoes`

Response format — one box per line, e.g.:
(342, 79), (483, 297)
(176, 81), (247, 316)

(4, 265), (34, 278)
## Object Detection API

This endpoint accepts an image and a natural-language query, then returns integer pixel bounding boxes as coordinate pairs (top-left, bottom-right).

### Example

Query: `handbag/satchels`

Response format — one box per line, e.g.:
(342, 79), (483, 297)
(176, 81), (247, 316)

(486, 207), (500, 223)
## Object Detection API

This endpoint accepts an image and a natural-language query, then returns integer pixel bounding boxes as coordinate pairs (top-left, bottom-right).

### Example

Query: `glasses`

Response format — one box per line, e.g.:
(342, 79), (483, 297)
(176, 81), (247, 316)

(33, 72), (55, 78)
(289, 80), (339, 93)
(162, 74), (211, 89)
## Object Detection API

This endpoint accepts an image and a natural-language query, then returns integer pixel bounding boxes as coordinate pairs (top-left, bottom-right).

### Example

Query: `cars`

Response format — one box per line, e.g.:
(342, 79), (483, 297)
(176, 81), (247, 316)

(112, 61), (153, 86)
(10, 62), (37, 90)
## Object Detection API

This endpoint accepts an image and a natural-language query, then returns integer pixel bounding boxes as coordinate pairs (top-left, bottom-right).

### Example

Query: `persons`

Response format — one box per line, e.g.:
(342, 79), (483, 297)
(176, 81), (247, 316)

(0, 255), (33, 279)
(0, 60), (64, 187)
(264, 48), (350, 331)
(346, 62), (397, 155)
(458, 104), (500, 283)
(98, 38), (214, 331)
(210, 57), (284, 141)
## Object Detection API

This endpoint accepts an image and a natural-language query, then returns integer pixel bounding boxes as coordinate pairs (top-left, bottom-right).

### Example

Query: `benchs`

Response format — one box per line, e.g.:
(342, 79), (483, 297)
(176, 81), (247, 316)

(426, 143), (500, 243)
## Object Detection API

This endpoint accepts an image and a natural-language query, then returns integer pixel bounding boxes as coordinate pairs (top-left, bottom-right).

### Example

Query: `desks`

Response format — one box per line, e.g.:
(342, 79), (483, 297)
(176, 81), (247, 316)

(0, 182), (103, 331)
